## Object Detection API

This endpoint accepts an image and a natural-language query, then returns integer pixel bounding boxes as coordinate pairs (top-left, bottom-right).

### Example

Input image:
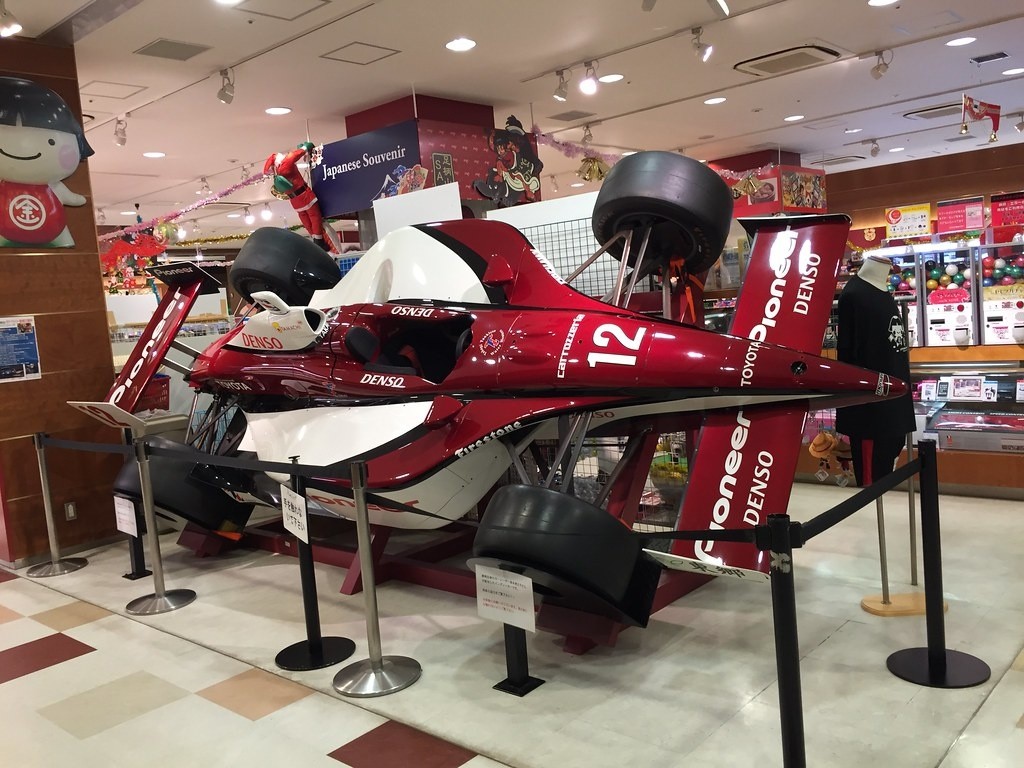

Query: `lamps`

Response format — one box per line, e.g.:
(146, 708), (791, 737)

(97, 209), (106, 224)
(871, 51), (888, 79)
(217, 66), (236, 104)
(581, 127), (594, 146)
(115, 119), (126, 145)
(691, 26), (713, 61)
(199, 177), (211, 195)
(1014, 115), (1024, 131)
(550, 177), (558, 192)
(241, 168), (250, 182)
(870, 141), (880, 157)
(553, 71), (569, 101)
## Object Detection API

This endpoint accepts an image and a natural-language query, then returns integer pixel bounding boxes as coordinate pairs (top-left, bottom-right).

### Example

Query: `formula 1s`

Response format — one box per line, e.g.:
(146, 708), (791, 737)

(59, 150), (918, 630)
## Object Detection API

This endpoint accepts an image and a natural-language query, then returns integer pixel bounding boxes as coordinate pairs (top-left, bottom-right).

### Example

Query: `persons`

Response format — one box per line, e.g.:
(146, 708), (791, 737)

(595, 437), (633, 488)
(834, 256), (916, 490)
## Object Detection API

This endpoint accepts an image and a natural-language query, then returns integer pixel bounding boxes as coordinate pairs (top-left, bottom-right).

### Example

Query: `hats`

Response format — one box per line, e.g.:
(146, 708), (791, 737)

(808, 433), (834, 458)
(831, 438), (852, 460)
